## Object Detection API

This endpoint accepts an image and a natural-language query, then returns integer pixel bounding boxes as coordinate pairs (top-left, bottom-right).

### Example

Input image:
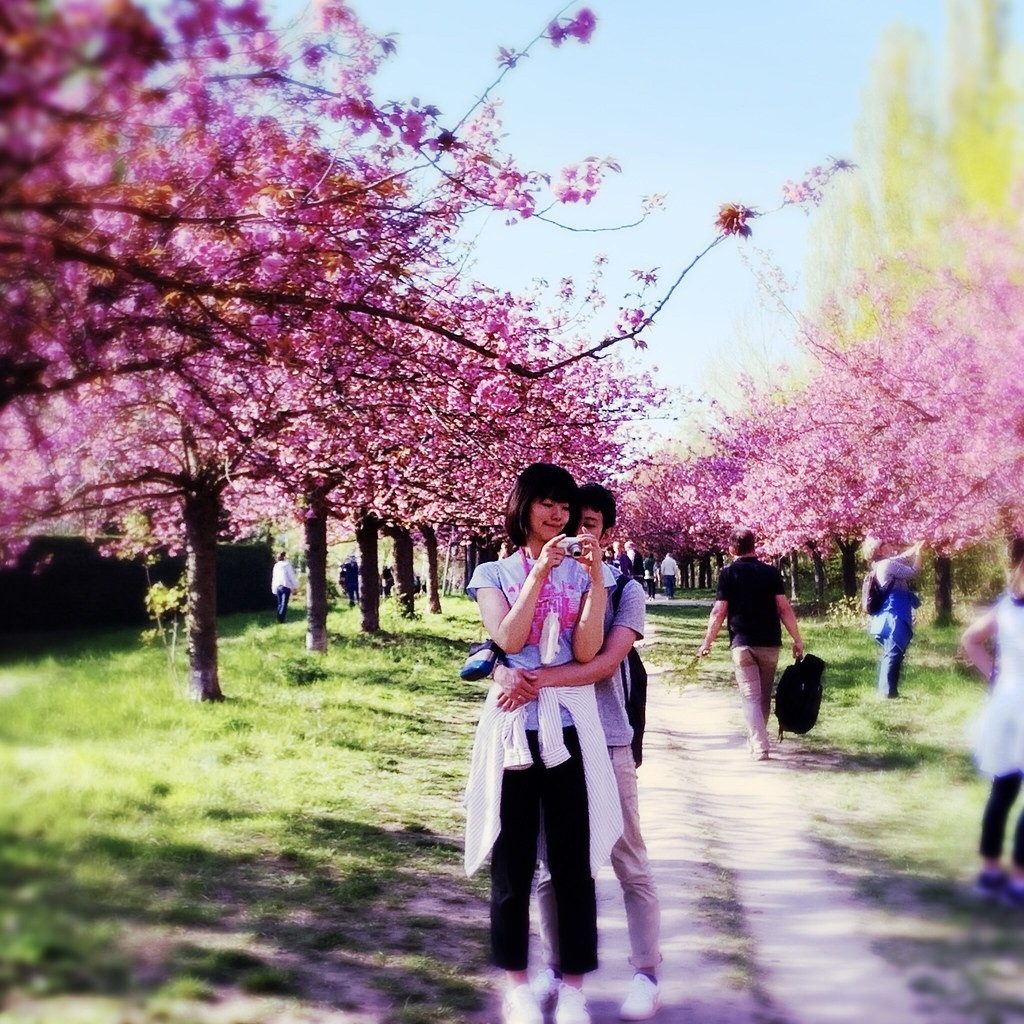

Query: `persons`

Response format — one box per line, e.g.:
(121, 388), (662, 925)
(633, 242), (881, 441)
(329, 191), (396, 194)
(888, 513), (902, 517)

(695, 530), (804, 762)
(962, 536), (1024, 906)
(864, 535), (927, 698)
(497, 542), (509, 560)
(467, 461), (617, 1024)
(340, 555), (450, 607)
(601, 546), (619, 570)
(661, 552), (679, 599)
(619, 539), (645, 591)
(485, 482), (664, 1021)
(644, 553), (657, 601)
(272, 553), (299, 623)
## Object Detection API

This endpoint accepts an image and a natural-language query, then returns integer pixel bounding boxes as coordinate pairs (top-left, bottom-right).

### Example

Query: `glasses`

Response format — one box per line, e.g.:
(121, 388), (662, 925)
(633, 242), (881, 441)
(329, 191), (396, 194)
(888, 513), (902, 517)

(879, 538), (887, 549)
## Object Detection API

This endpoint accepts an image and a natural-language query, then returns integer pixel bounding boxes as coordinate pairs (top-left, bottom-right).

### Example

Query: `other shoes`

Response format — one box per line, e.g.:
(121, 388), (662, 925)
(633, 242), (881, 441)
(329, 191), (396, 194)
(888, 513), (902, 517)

(755, 751), (768, 761)
(973, 867), (1024, 906)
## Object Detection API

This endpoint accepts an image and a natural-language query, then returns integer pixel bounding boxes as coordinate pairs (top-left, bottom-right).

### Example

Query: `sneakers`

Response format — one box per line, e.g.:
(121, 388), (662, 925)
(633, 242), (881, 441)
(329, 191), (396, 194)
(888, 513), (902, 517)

(502, 984), (544, 1024)
(619, 973), (662, 1020)
(530, 968), (562, 1012)
(555, 983), (591, 1024)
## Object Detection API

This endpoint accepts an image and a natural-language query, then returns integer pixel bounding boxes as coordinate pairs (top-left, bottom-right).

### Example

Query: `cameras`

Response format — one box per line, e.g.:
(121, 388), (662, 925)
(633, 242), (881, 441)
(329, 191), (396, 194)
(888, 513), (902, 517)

(556, 536), (585, 559)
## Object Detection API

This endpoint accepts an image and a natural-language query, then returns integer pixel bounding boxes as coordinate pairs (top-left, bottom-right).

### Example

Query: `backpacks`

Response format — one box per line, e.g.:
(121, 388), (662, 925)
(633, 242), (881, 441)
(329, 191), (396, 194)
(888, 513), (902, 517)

(862, 567), (896, 614)
(612, 574), (647, 769)
(774, 654), (826, 743)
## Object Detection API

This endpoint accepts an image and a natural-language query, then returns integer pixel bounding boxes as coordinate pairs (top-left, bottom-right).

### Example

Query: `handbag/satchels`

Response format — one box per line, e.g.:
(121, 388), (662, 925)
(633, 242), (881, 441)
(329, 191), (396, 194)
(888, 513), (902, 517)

(460, 639), (510, 681)
